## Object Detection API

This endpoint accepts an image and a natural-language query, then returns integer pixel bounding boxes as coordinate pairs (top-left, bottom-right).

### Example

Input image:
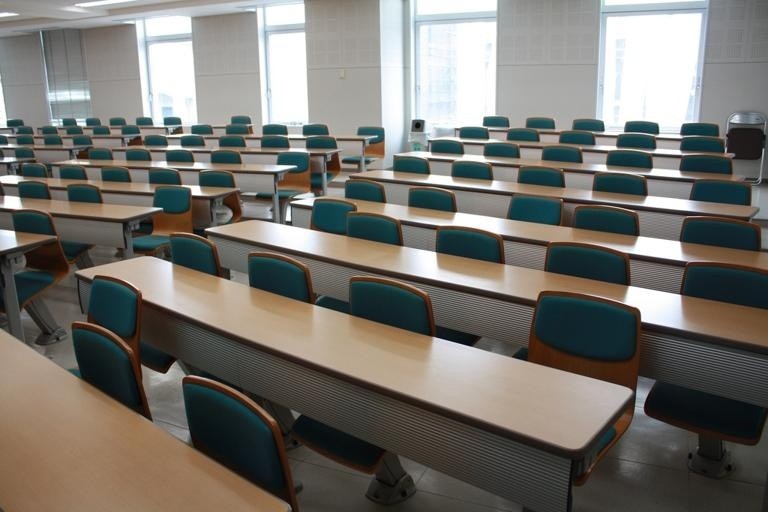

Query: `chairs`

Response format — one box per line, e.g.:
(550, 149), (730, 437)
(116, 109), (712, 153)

(1, 115), (768, 512)
